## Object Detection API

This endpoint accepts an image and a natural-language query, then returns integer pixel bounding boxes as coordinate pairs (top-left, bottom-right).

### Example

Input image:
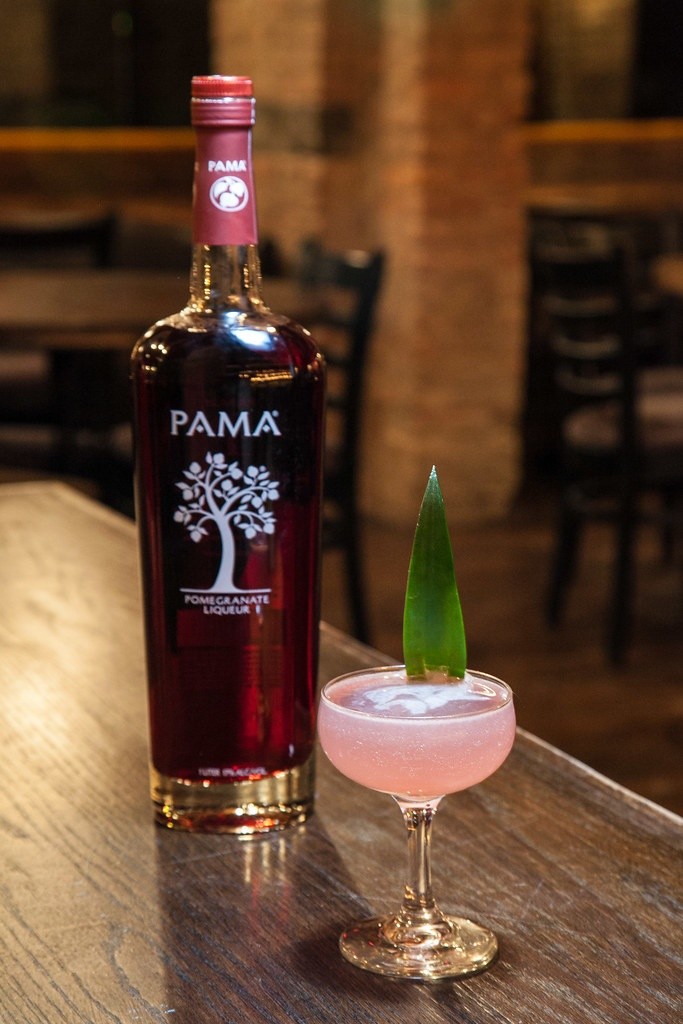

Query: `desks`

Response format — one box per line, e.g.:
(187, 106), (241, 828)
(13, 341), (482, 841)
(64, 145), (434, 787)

(0, 476), (683, 1024)
(0, 270), (322, 350)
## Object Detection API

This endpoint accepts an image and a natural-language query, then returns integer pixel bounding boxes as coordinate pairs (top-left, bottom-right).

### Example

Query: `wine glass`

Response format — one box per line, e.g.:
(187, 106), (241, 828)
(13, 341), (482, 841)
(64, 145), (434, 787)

(316, 663), (518, 982)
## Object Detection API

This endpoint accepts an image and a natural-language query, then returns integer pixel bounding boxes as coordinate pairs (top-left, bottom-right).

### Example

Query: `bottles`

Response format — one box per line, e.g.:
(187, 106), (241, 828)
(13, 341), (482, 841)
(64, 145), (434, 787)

(129, 73), (329, 843)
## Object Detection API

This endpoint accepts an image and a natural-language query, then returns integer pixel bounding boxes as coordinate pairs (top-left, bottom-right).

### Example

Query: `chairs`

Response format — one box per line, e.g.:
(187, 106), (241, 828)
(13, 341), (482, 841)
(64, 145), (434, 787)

(0, 200), (391, 644)
(515, 200), (683, 662)
